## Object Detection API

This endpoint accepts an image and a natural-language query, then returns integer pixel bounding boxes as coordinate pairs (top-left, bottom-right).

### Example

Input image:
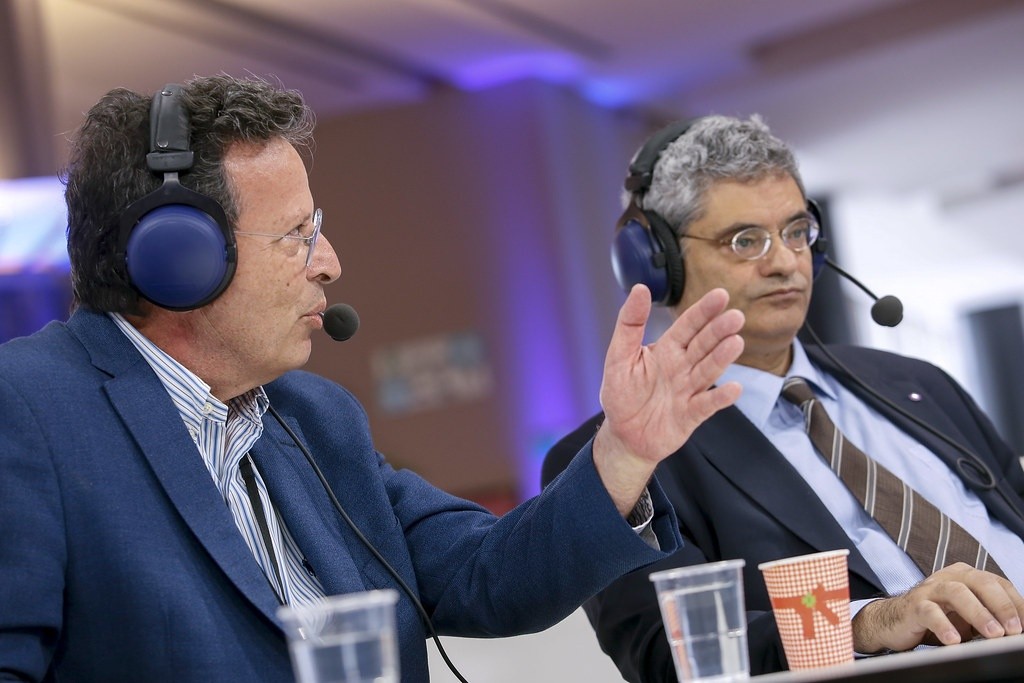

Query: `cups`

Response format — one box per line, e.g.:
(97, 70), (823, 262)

(758, 548), (854, 670)
(649, 559), (750, 683)
(274, 587), (400, 683)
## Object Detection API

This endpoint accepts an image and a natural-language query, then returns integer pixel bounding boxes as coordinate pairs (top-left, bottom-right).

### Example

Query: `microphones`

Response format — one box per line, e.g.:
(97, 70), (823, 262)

(319, 304), (360, 341)
(825, 254), (904, 328)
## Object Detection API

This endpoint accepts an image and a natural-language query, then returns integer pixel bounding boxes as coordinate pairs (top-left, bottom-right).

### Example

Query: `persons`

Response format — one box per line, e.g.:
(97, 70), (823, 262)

(538, 114), (1024, 683)
(0, 75), (744, 683)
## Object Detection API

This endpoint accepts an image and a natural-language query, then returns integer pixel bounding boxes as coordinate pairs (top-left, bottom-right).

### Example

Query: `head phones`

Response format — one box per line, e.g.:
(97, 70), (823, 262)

(610, 121), (826, 306)
(121, 82), (238, 311)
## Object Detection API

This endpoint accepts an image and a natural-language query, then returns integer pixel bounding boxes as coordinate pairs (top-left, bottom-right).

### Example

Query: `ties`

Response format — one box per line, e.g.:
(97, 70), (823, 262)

(780, 377), (1013, 641)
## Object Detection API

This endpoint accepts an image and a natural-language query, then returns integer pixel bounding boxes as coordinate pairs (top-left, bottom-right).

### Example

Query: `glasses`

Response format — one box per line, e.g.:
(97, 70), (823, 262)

(233, 208), (324, 266)
(678, 218), (820, 259)
